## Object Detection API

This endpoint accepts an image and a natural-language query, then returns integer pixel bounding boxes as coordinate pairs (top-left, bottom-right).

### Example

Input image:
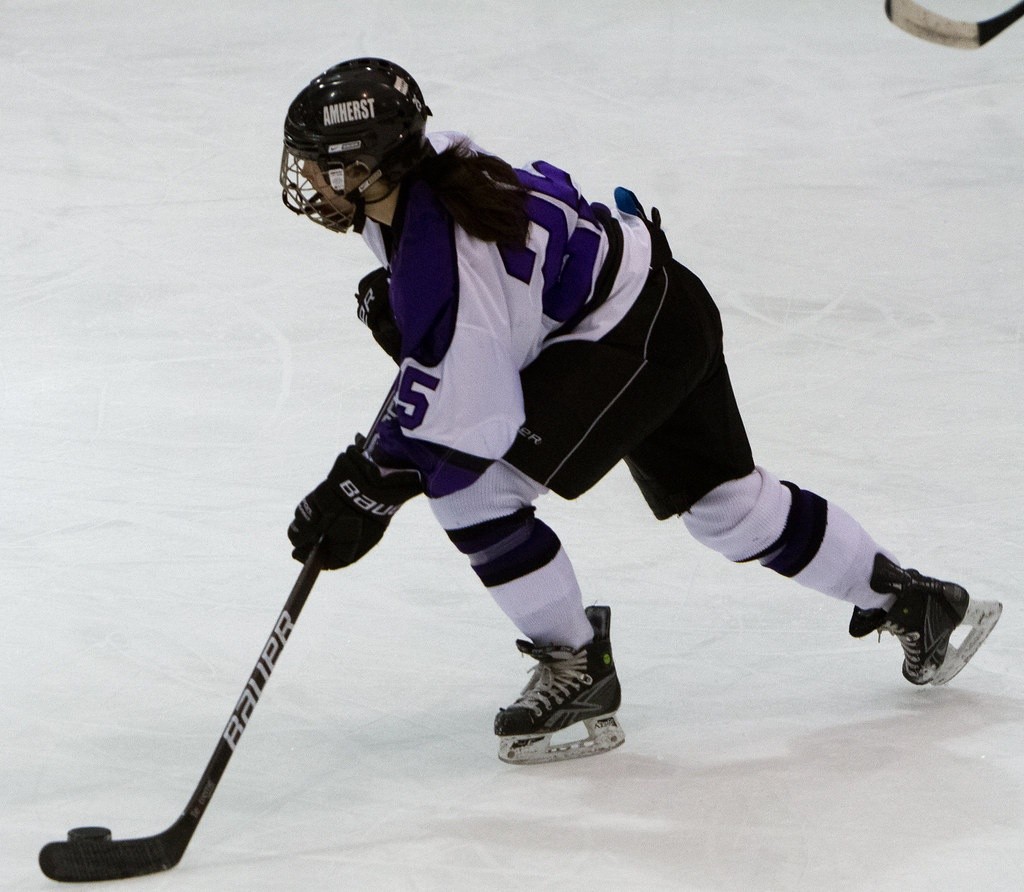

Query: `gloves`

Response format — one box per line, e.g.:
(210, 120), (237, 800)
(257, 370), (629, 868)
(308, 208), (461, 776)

(287, 433), (423, 570)
(354, 268), (401, 369)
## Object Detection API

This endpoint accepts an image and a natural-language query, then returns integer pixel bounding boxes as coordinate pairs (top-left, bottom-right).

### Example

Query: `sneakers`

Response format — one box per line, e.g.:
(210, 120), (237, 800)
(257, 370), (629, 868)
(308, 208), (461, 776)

(494, 605), (621, 736)
(849, 553), (969, 685)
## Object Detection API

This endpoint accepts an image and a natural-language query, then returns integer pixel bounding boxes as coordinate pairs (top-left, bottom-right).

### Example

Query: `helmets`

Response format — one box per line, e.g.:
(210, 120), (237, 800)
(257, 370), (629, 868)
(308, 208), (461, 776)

(281, 58), (433, 232)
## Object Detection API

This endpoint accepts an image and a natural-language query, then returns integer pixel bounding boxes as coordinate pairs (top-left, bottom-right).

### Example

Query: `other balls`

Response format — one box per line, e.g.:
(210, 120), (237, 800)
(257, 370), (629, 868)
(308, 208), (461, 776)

(66, 825), (113, 843)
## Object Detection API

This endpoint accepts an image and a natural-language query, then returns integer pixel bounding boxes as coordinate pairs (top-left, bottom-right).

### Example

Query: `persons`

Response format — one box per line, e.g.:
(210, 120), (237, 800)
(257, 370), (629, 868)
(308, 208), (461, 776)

(279, 58), (1004, 765)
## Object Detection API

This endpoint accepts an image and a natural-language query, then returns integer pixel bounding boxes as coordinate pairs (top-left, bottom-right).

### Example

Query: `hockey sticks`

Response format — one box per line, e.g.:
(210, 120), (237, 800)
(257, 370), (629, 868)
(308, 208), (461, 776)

(883, 0), (1024, 52)
(37, 373), (404, 884)
